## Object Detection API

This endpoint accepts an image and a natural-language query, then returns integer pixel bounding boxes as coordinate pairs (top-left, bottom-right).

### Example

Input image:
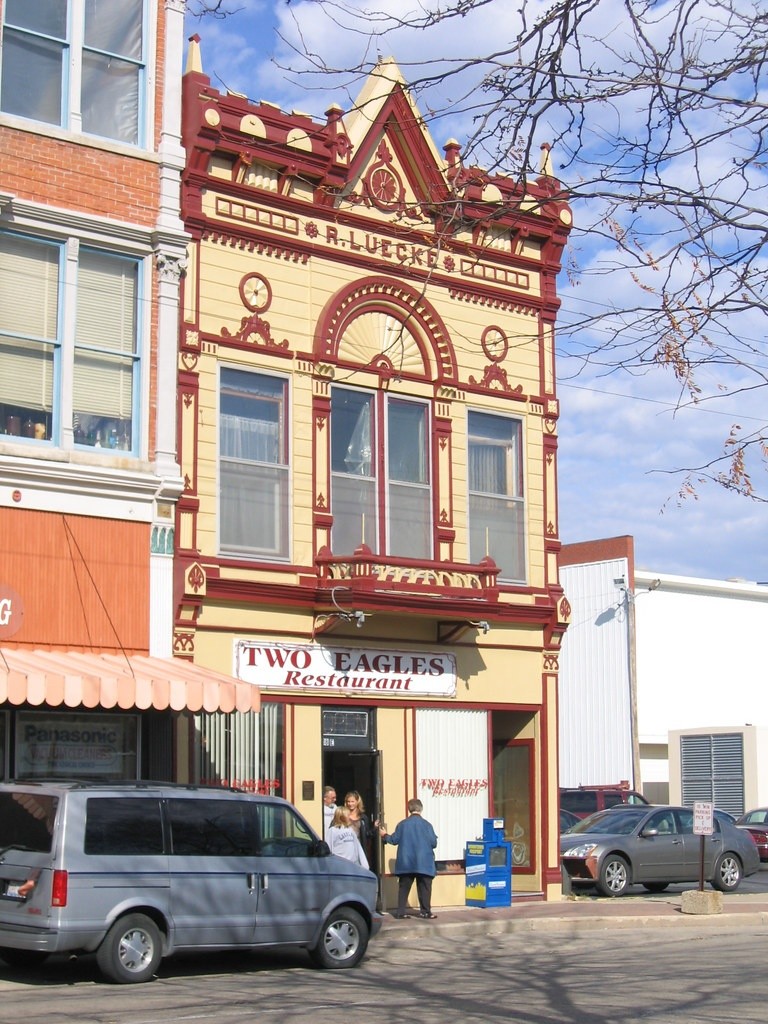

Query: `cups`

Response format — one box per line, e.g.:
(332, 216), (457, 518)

(6, 415), (21, 436)
(34, 423), (46, 439)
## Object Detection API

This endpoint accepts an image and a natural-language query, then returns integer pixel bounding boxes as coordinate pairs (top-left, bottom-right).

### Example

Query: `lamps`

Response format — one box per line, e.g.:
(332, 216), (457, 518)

(629, 579), (661, 602)
(354, 610), (365, 628)
(464, 616), (489, 634)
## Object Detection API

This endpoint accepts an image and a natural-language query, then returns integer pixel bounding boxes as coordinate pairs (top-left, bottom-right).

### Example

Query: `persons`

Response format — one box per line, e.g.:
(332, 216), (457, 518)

(344, 791), (384, 916)
(326, 807), (369, 870)
(324, 787), (337, 836)
(380, 799), (438, 919)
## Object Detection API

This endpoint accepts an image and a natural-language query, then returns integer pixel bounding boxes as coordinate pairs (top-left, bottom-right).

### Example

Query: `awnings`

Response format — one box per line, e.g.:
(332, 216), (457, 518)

(0, 648), (260, 717)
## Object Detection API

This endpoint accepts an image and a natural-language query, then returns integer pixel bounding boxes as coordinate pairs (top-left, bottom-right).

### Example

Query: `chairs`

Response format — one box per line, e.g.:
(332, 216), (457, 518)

(655, 819), (671, 836)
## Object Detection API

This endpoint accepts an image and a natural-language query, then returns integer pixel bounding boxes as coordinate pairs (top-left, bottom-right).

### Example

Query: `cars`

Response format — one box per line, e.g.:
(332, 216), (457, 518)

(560, 809), (585, 833)
(560, 805), (760, 897)
(735, 807), (768, 859)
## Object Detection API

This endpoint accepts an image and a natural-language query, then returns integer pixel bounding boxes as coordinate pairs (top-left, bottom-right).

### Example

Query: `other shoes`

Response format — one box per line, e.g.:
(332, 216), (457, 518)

(396, 912), (407, 918)
(420, 913), (437, 918)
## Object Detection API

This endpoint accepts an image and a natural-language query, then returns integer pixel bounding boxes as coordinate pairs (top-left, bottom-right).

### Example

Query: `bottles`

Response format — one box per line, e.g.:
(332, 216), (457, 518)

(86, 428), (96, 446)
(74, 424), (84, 444)
(22, 416), (35, 437)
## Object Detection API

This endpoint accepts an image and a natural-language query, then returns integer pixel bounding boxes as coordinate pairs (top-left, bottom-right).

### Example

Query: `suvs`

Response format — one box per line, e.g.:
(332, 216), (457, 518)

(0, 777), (385, 986)
(559, 783), (654, 819)
